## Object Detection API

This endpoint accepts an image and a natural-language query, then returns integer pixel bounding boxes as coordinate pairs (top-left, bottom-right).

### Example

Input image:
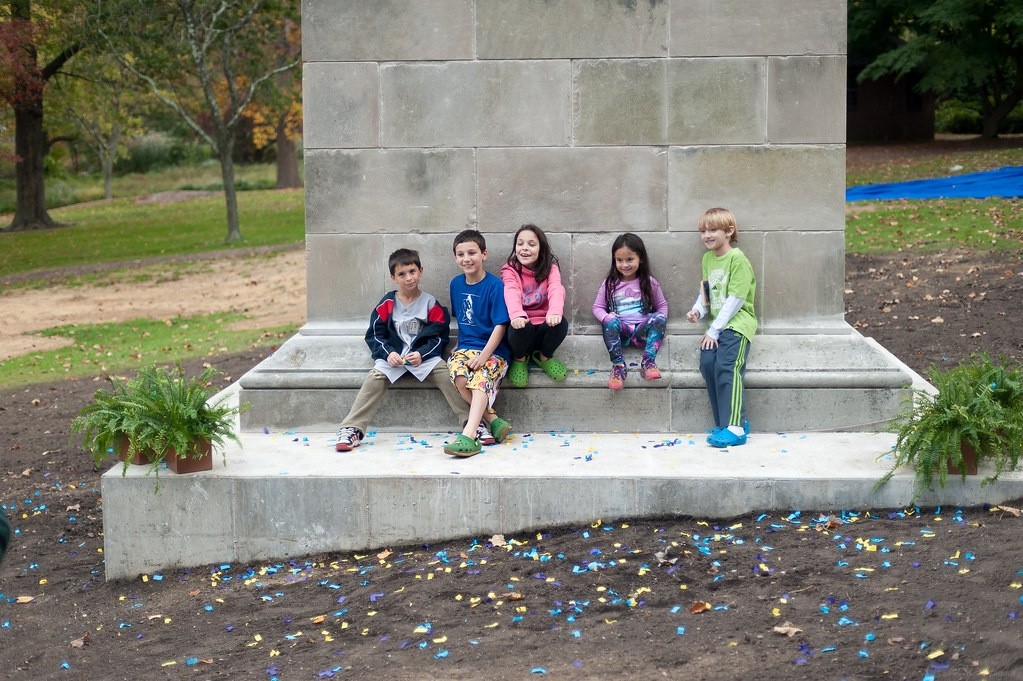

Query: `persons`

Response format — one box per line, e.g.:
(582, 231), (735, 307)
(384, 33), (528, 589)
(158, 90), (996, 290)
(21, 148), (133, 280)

(335, 248), (496, 452)
(592, 233), (668, 390)
(499, 224), (570, 386)
(685, 209), (757, 447)
(443, 230), (512, 457)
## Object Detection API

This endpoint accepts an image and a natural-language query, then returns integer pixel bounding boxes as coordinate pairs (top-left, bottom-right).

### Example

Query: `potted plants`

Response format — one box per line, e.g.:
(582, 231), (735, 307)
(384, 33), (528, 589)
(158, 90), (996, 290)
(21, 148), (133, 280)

(873, 351), (1023, 501)
(70, 361), (254, 495)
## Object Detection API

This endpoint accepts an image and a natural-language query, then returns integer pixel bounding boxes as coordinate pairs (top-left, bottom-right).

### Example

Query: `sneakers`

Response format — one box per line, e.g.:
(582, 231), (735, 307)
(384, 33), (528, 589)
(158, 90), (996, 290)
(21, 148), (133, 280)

(475, 421), (495, 444)
(607, 362), (627, 390)
(640, 359), (662, 381)
(335, 427), (360, 451)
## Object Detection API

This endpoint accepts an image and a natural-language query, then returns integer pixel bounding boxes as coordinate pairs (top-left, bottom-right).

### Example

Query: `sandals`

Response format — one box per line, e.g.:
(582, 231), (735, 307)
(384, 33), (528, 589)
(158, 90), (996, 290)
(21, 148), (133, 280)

(490, 418), (512, 444)
(706, 419), (751, 444)
(532, 351), (569, 382)
(444, 435), (481, 457)
(508, 354), (531, 388)
(711, 428), (747, 447)
